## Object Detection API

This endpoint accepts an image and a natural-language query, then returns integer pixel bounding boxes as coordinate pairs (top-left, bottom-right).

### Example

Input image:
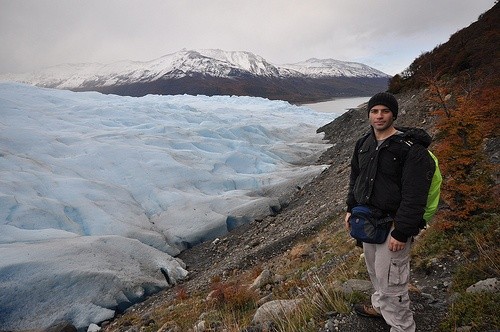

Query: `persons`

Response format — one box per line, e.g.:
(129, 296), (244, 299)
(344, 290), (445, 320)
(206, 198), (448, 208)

(344, 92), (436, 331)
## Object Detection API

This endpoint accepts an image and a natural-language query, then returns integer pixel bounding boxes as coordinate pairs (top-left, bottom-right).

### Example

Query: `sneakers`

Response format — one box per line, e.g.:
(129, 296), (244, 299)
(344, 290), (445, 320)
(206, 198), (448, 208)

(355, 302), (383, 318)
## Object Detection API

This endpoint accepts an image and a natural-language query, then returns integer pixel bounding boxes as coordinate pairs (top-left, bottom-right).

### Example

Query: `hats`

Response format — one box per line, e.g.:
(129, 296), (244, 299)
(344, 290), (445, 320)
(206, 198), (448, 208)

(368, 92), (398, 121)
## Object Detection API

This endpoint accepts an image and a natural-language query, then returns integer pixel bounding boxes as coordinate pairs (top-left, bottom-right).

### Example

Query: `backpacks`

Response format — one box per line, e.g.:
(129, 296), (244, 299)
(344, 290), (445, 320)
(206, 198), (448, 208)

(359, 135), (442, 229)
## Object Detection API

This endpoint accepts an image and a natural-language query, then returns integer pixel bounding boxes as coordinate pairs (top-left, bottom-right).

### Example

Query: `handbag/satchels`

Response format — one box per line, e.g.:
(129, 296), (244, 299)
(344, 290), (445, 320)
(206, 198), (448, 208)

(347, 205), (392, 244)
